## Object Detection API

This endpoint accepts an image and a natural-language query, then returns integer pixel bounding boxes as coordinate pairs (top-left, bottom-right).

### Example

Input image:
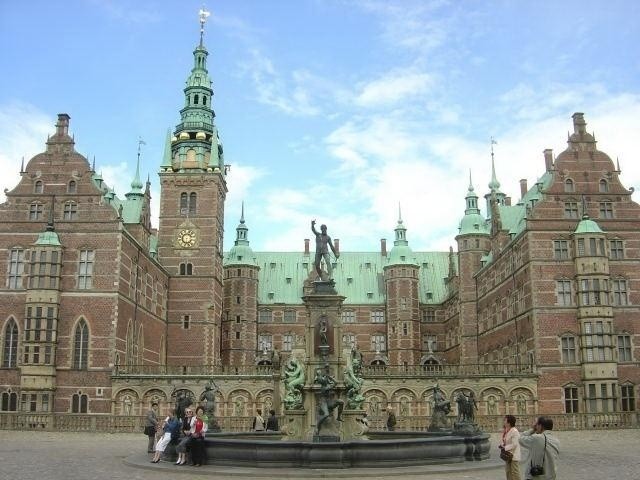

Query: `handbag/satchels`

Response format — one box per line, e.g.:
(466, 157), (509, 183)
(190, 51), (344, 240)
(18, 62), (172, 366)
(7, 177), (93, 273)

(500, 449), (513, 463)
(144, 426), (155, 436)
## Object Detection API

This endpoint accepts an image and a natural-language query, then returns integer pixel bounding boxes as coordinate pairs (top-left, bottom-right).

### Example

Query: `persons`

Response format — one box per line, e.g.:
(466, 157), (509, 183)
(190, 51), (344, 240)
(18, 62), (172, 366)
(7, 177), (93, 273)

(149, 406), (208, 467)
(455, 392), (467, 422)
(266, 409), (280, 431)
(310, 219), (339, 283)
(146, 402), (158, 453)
(499, 415), (521, 480)
(518, 415), (563, 480)
(253, 408), (265, 431)
(466, 392), (478, 421)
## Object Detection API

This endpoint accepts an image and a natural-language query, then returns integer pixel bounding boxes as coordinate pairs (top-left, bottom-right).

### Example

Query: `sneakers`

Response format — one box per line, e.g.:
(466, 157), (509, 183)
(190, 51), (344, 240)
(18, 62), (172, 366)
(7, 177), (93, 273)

(190, 463), (200, 466)
(151, 458), (159, 462)
(174, 460), (186, 465)
